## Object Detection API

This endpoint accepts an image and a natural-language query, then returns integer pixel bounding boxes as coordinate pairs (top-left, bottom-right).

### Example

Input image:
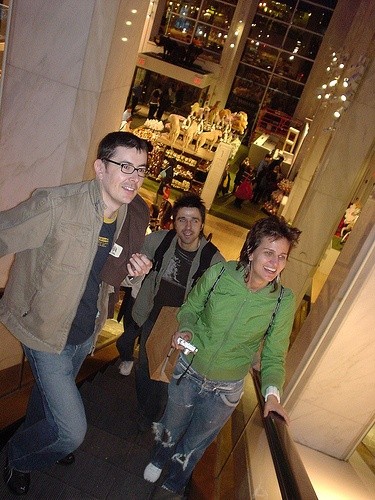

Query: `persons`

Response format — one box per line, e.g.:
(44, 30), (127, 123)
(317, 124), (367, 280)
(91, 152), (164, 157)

(231, 152), (286, 209)
(144, 214), (303, 498)
(119, 79), (183, 131)
(115, 157), (226, 431)
(0, 131), (153, 495)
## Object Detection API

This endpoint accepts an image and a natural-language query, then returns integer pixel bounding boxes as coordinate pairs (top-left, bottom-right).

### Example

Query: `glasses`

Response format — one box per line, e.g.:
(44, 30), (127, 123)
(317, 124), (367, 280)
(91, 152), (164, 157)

(102, 158), (151, 178)
(173, 219), (202, 226)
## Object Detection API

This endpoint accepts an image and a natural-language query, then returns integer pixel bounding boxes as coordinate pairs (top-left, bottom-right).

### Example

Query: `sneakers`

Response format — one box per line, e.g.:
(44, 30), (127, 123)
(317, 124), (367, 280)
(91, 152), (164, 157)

(161, 485), (175, 494)
(57, 454), (74, 465)
(144, 462), (162, 483)
(5, 451), (30, 495)
(119, 361), (134, 375)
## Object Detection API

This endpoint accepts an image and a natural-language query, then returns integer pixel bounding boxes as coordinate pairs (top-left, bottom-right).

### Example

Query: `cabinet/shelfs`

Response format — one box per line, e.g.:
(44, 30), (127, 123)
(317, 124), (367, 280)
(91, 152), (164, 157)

(247, 107), (309, 190)
(132, 124), (233, 211)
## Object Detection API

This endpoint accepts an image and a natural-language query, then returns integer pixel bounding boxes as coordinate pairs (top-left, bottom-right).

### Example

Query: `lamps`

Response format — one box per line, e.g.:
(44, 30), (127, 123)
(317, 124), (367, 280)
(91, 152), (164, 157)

(334, 55), (372, 117)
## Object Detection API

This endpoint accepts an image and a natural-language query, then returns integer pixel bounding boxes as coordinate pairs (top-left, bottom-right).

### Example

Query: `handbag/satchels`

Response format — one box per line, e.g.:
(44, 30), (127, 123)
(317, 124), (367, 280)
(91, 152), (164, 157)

(144, 306), (182, 384)
(156, 166), (172, 182)
(234, 183), (254, 200)
